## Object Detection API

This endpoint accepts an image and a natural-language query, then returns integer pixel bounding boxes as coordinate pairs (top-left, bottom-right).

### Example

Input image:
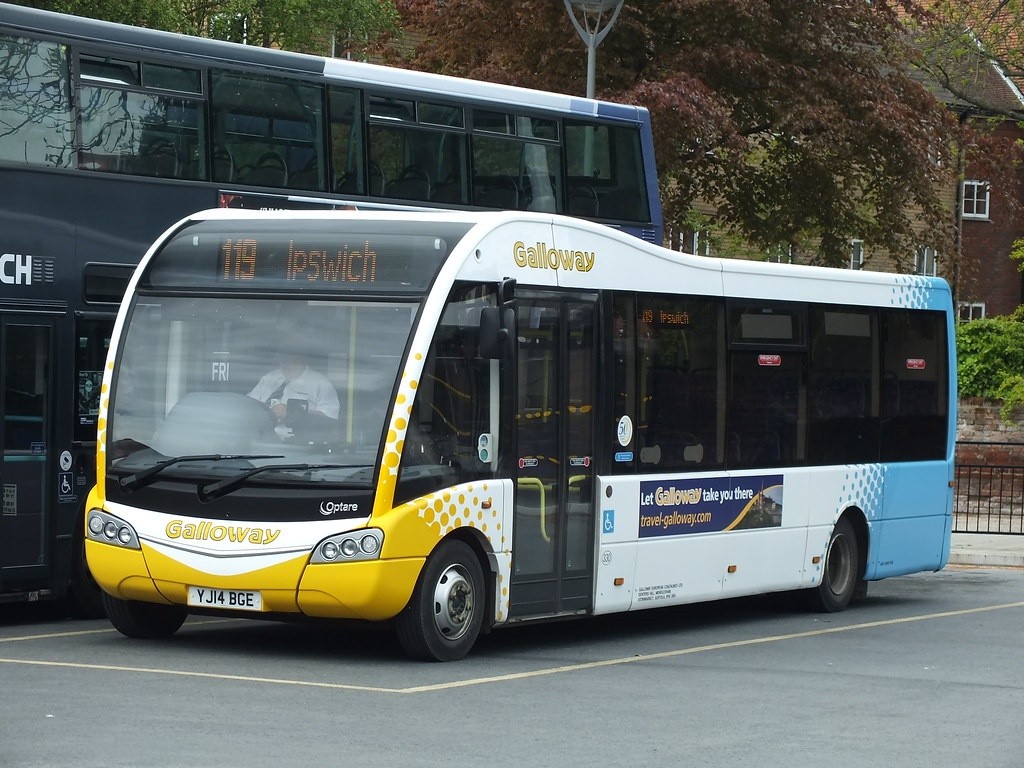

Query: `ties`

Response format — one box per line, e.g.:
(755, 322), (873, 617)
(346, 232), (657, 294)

(265, 378), (290, 406)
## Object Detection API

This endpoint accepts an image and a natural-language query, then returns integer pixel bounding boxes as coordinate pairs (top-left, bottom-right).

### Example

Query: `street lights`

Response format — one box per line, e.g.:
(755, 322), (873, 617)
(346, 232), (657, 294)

(560, 0), (625, 177)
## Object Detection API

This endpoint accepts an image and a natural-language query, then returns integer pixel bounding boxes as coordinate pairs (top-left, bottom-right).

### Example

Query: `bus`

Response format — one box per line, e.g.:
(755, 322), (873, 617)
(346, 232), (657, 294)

(81, 200), (959, 665)
(0, 0), (665, 619)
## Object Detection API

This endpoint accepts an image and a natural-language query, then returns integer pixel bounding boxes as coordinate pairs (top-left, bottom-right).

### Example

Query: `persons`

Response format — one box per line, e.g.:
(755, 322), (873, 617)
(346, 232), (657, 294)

(243, 335), (339, 445)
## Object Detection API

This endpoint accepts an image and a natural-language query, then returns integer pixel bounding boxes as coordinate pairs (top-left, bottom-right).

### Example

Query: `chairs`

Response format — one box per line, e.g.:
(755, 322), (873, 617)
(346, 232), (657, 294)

(82, 135), (599, 216)
